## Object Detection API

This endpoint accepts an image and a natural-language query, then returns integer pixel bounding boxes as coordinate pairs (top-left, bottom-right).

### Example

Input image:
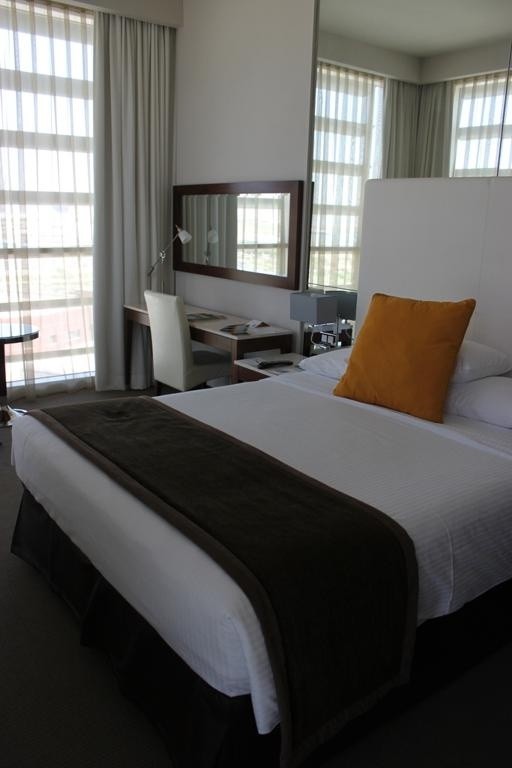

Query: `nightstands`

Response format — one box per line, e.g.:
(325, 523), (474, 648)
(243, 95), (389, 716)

(232, 350), (307, 384)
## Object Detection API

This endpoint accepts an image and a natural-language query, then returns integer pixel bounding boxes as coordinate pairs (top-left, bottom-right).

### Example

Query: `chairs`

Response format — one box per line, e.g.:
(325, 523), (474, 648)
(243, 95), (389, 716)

(142, 289), (245, 396)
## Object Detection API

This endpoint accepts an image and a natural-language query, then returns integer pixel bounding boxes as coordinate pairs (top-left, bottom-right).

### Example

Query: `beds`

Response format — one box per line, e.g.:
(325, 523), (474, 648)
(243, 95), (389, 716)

(8, 174), (512, 768)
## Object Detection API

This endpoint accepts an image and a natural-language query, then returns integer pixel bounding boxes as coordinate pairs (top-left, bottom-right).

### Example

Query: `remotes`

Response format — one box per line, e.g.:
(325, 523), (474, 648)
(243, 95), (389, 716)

(258, 360), (293, 369)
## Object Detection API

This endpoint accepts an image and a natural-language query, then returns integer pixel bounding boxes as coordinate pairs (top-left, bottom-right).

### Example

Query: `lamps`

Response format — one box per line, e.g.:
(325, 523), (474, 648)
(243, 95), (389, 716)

(147, 223), (192, 293)
(289, 291), (340, 352)
(199, 222), (219, 264)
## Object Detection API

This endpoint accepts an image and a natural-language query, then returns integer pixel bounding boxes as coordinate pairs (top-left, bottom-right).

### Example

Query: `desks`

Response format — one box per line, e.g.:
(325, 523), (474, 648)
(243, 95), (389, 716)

(121, 303), (293, 393)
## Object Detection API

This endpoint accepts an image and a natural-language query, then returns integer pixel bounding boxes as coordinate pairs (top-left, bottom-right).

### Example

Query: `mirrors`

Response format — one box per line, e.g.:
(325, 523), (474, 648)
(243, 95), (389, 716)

(171, 179), (302, 291)
(301, 0), (511, 321)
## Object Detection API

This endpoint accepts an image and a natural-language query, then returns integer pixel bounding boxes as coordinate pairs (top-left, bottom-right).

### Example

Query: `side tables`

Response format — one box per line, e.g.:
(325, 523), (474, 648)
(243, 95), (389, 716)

(1, 323), (41, 430)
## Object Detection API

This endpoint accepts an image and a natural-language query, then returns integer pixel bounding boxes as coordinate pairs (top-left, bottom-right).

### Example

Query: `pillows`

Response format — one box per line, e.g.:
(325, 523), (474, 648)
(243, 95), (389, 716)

(295, 288), (511, 424)
